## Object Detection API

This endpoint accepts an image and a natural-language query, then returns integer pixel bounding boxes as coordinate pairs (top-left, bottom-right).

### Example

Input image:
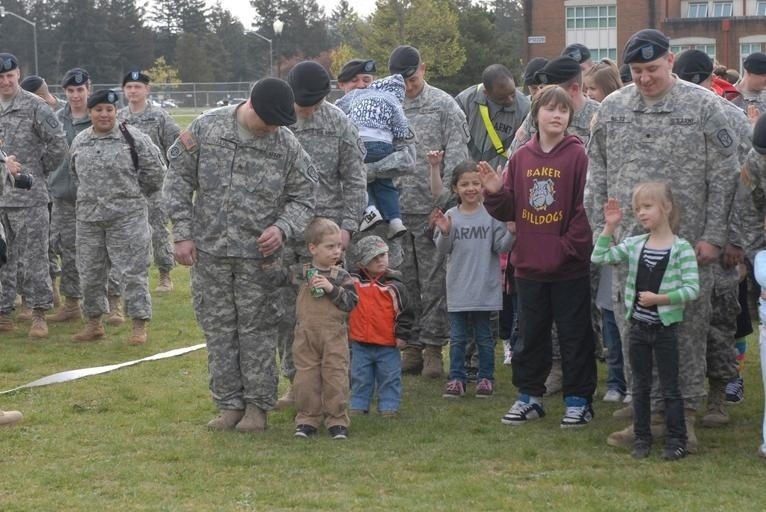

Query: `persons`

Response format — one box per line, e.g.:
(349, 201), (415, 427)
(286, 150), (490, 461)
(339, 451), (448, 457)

(275, 45), (471, 442)
(2, 52), (182, 344)
(160, 76), (320, 429)
(434, 29), (766, 460)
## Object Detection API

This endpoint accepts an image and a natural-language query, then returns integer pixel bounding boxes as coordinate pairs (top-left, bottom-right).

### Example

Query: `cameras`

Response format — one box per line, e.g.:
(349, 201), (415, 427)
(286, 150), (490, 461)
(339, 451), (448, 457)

(15, 173), (33, 190)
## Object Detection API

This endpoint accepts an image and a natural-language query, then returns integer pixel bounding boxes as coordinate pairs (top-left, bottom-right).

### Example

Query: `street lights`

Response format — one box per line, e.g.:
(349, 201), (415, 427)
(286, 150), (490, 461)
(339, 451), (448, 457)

(0, 6), (38, 77)
(241, 28), (273, 77)
(271, 19), (285, 80)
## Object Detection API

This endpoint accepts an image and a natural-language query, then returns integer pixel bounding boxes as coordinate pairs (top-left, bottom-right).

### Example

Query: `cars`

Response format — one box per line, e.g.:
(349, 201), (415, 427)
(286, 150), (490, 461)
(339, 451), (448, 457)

(217, 97), (246, 107)
(146, 95), (181, 110)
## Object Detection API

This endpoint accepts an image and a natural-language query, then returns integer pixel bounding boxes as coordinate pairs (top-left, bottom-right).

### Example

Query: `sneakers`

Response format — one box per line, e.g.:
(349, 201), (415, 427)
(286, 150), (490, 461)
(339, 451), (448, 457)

(208, 392), (396, 439)
(603, 378), (744, 459)
(387, 222), (407, 240)
(360, 209), (383, 231)
(442, 350), (599, 427)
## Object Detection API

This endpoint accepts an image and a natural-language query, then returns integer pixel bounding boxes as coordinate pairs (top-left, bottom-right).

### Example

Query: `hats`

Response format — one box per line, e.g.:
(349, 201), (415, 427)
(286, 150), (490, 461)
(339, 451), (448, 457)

(20, 76), (43, 92)
(356, 236), (389, 265)
(250, 61), (331, 124)
(744, 54), (766, 74)
(523, 43), (590, 84)
(620, 29), (713, 84)
(338, 46), (420, 81)
(752, 113), (766, 153)
(62, 68), (150, 107)
(0, 53), (18, 72)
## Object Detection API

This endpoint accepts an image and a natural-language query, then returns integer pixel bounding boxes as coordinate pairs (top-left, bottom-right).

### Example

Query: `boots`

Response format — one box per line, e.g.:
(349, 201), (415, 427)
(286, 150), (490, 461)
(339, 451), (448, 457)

(400, 344), (443, 378)
(0, 276), (148, 345)
(155, 270), (173, 292)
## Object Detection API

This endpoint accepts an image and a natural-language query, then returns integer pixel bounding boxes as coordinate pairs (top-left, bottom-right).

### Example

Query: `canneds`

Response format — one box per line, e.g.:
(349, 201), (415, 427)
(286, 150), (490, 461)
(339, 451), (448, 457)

(306, 268), (325, 298)
